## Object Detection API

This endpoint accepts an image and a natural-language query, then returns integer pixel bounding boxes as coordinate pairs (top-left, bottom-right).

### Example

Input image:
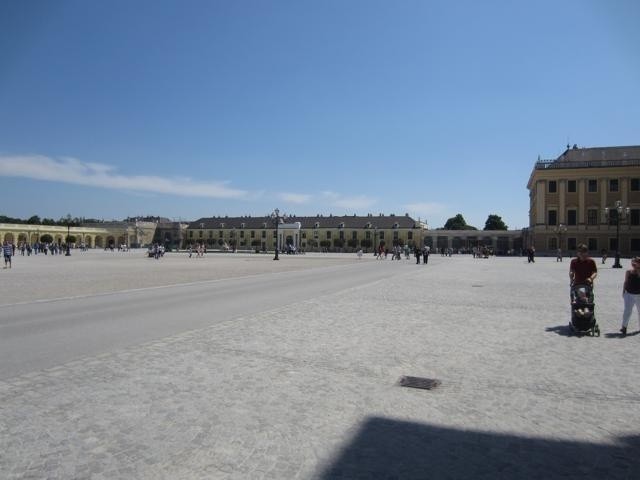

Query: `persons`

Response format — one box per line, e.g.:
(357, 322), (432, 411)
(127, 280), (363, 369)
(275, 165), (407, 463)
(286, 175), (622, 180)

(104, 242), (129, 252)
(2, 240), (13, 269)
(619, 256), (640, 334)
(572, 287), (592, 317)
(557, 246), (563, 262)
(148, 241), (165, 259)
(0, 241), (90, 257)
(189, 242), (207, 258)
(355, 242), (535, 265)
(568, 243), (596, 293)
(287, 242), (306, 256)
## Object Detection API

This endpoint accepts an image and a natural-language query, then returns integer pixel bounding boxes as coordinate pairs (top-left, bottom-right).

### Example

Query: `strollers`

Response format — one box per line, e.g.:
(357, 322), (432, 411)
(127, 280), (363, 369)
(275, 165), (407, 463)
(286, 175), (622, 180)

(569, 277), (600, 339)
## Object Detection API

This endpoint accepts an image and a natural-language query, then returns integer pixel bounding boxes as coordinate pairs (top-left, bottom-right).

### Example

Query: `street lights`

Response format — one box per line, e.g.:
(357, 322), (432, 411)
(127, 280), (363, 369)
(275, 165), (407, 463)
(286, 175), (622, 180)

(604, 200), (631, 269)
(553, 223), (568, 262)
(272, 207), (280, 261)
(65, 217), (72, 256)
